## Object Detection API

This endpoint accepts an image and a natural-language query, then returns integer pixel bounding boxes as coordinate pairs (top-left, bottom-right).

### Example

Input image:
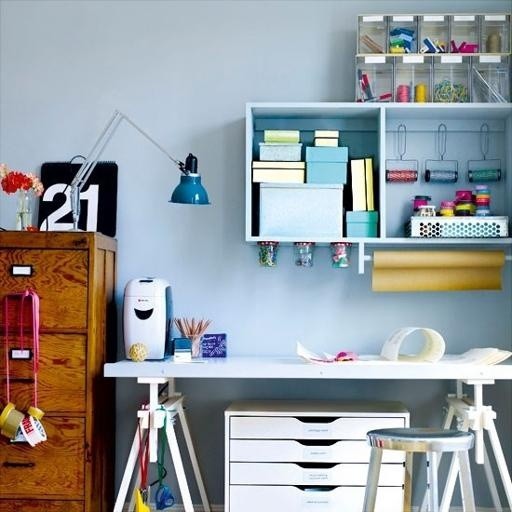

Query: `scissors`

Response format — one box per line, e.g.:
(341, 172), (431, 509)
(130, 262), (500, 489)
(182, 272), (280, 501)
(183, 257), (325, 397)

(155, 485), (175, 512)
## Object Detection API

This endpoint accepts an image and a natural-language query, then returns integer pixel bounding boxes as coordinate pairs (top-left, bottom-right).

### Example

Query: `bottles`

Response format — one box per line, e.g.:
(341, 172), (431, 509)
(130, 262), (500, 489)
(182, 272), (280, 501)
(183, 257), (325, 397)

(487, 30), (502, 54)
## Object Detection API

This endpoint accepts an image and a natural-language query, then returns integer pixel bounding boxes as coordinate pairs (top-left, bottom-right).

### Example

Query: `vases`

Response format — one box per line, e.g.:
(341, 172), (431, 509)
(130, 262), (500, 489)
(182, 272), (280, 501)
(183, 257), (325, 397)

(15, 196), (33, 229)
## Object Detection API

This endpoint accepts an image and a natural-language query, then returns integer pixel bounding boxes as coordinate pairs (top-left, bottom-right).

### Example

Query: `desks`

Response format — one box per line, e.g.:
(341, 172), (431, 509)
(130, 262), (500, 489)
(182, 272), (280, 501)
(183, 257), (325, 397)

(102, 355), (512, 512)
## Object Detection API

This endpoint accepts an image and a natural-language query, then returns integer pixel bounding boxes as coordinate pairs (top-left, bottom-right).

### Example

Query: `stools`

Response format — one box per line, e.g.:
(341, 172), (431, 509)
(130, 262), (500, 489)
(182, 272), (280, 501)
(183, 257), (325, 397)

(364, 428), (476, 511)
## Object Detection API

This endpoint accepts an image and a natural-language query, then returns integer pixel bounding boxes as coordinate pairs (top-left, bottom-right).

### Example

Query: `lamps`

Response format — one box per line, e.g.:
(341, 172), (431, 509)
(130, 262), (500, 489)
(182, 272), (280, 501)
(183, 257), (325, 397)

(68, 110), (209, 233)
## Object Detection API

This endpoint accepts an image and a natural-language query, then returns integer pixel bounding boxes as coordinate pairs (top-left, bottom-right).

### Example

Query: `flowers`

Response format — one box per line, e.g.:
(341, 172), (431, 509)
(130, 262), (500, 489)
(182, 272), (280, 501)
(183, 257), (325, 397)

(1, 165), (44, 195)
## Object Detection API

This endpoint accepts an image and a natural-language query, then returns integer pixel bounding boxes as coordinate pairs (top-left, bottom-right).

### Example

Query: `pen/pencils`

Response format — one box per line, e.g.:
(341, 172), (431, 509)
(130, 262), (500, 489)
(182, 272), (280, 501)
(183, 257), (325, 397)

(174, 317), (213, 357)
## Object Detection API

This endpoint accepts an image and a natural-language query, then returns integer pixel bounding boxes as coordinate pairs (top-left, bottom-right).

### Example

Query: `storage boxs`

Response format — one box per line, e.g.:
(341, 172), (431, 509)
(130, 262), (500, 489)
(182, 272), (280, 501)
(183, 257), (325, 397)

(353, 11), (512, 102)
(223, 399), (411, 512)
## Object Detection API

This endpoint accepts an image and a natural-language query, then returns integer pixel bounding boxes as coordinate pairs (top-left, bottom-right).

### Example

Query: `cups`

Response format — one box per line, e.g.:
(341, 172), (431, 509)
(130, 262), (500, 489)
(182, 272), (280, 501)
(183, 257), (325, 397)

(181, 335), (203, 358)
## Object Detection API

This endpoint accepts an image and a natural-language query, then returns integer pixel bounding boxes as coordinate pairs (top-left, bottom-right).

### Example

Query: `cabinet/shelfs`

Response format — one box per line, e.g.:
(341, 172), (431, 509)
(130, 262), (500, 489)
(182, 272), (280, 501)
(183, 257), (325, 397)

(0, 231), (118, 511)
(245, 102), (512, 248)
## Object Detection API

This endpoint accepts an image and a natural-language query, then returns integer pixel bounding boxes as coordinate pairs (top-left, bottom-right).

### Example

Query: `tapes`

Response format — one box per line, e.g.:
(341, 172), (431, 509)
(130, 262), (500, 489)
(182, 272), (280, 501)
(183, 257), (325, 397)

(0, 409), (25, 439)
(0, 403), (15, 427)
(20, 415), (47, 447)
(27, 406), (44, 420)
